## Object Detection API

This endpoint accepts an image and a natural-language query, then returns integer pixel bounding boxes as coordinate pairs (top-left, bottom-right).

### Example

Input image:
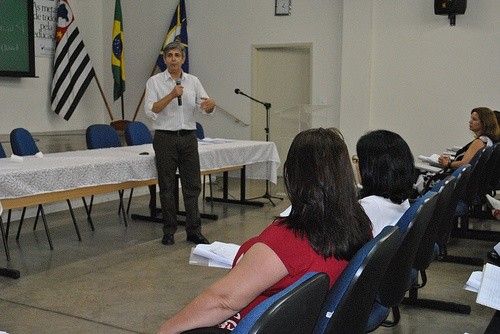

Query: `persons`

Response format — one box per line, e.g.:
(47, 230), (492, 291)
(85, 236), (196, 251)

(350, 130), (420, 241)
(154, 127), (374, 334)
(413, 106), (500, 201)
(144, 41), (215, 245)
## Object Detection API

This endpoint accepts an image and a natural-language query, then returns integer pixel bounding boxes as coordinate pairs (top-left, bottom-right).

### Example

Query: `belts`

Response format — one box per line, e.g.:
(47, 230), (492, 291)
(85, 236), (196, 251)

(155, 129), (196, 136)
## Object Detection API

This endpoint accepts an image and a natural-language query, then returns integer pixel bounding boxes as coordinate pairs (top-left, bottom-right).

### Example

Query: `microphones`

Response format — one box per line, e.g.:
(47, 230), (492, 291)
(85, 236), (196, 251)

(235, 89), (244, 95)
(175, 78), (182, 106)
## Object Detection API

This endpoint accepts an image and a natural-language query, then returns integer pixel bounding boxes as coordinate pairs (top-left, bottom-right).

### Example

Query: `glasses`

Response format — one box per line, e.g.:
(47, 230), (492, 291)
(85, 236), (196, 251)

(352, 155), (358, 164)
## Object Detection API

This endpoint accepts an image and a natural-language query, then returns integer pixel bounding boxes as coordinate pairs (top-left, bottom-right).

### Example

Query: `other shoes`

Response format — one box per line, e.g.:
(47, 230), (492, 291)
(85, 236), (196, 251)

(487, 251), (500, 267)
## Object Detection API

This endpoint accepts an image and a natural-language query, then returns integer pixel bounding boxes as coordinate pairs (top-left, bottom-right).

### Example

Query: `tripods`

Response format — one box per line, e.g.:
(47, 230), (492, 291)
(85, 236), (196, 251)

(244, 93), (283, 206)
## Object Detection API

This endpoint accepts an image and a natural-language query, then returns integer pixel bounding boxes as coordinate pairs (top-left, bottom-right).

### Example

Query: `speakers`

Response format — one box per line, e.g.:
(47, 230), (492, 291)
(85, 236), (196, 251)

(434, 0), (467, 14)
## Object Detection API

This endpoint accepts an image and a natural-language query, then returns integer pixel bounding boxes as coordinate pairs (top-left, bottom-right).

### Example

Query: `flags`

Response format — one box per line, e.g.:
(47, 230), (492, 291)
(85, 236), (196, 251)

(110, 0), (127, 102)
(156, 0), (189, 75)
(50, 0), (95, 122)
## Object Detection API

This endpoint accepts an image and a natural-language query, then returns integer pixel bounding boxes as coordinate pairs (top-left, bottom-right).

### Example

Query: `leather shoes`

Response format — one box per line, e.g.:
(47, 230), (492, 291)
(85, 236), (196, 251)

(187, 233), (210, 244)
(162, 234), (175, 245)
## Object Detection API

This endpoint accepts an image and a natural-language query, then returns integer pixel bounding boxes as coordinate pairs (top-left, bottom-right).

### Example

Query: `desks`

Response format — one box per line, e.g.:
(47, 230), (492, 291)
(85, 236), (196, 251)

(0, 136), (282, 262)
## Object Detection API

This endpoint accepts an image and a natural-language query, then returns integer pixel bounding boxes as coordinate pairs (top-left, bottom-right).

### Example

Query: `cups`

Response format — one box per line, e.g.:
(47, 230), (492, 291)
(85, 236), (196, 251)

(9, 153), (23, 163)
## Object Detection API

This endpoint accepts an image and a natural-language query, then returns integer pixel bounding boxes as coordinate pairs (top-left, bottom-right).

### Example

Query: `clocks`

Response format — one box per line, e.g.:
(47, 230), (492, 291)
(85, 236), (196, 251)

(275, 0), (292, 16)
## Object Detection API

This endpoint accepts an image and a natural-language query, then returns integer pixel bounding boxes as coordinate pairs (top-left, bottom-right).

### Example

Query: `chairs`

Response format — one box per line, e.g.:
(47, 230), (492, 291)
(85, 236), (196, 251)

(85, 124), (129, 228)
(231, 141), (500, 334)
(10, 128), (82, 250)
(124, 120), (158, 214)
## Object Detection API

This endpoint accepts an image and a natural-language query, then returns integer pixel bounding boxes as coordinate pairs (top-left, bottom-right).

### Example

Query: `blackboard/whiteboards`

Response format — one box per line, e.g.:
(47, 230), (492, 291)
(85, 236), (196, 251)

(0, 0), (40, 78)
